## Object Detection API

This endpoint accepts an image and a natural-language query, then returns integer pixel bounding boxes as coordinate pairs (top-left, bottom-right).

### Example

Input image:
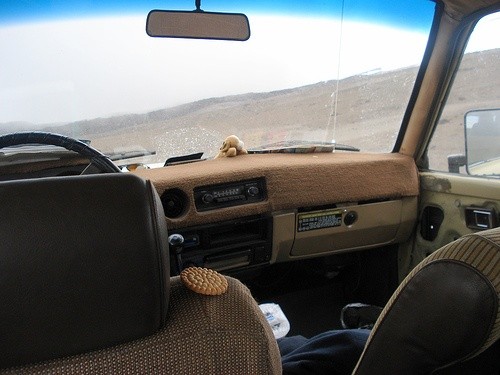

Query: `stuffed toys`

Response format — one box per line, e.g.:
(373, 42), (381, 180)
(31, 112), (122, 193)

(214, 135), (250, 160)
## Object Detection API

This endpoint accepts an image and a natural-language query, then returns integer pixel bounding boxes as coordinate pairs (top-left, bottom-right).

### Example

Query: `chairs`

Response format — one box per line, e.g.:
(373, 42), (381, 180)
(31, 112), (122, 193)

(350, 225), (500, 374)
(1, 174), (282, 375)
